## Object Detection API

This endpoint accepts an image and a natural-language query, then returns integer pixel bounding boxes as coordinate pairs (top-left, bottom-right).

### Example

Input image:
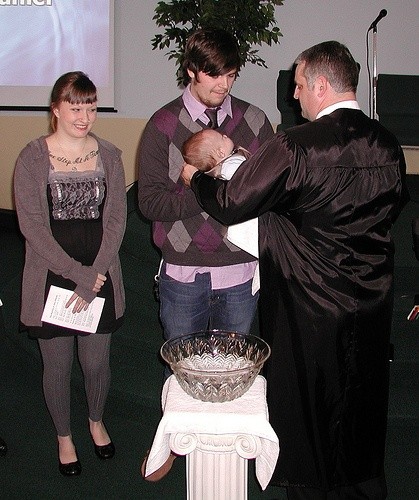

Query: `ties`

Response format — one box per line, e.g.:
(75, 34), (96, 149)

(204, 107), (221, 129)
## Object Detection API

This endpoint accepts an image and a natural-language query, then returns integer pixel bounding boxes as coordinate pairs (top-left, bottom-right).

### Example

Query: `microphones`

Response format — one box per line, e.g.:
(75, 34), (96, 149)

(368, 9), (388, 31)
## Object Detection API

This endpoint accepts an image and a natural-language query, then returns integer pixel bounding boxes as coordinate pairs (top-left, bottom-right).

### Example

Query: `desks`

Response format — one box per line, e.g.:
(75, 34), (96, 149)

(144, 374), (279, 500)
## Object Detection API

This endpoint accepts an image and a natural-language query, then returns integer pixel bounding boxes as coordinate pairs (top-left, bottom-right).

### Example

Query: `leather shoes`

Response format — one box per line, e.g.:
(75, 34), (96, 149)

(89, 420), (116, 460)
(58, 439), (81, 477)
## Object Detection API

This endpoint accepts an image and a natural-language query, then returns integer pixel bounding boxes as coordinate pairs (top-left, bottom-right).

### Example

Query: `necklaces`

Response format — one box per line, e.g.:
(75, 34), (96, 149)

(59, 136), (87, 172)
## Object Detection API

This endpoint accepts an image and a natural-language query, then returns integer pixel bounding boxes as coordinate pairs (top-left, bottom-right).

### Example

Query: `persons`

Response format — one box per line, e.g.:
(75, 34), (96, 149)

(136, 26), (277, 377)
(182, 129), (250, 181)
(12, 70), (127, 477)
(182, 40), (407, 500)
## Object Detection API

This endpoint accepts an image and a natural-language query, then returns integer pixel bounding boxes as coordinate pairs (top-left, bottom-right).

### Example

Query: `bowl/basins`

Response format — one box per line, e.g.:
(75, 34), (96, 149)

(160, 329), (272, 404)
